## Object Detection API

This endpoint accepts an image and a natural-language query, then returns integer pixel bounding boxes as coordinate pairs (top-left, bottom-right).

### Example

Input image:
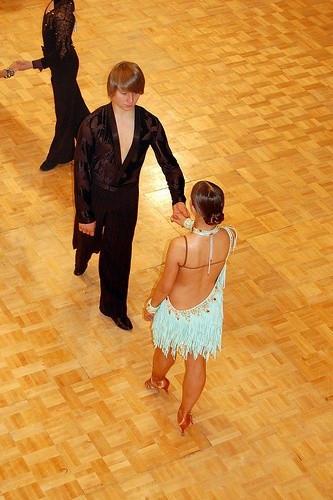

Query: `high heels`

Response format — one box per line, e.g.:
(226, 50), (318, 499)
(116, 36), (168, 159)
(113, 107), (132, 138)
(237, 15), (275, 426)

(177, 411), (193, 433)
(144, 376), (169, 392)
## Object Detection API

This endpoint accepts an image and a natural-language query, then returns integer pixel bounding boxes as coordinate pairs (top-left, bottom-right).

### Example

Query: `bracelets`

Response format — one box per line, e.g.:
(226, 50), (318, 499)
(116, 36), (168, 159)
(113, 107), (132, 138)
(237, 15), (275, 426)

(145, 298), (159, 314)
(4, 67), (15, 79)
(184, 218), (194, 230)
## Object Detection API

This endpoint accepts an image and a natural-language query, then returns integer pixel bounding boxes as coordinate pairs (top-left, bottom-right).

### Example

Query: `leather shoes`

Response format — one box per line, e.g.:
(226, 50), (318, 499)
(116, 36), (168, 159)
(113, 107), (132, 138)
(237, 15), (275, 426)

(74, 266), (87, 275)
(111, 315), (133, 330)
(40, 160), (57, 171)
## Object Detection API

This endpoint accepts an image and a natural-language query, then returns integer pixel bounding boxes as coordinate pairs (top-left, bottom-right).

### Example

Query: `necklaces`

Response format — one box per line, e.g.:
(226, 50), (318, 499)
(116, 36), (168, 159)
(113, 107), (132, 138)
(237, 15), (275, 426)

(192, 226), (220, 276)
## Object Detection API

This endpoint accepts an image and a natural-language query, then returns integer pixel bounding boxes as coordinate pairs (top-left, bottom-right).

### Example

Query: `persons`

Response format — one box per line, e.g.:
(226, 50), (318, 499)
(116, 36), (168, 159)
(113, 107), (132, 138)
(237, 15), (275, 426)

(72, 61), (190, 331)
(142, 180), (237, 436)
(16, 0), (91, 171)
